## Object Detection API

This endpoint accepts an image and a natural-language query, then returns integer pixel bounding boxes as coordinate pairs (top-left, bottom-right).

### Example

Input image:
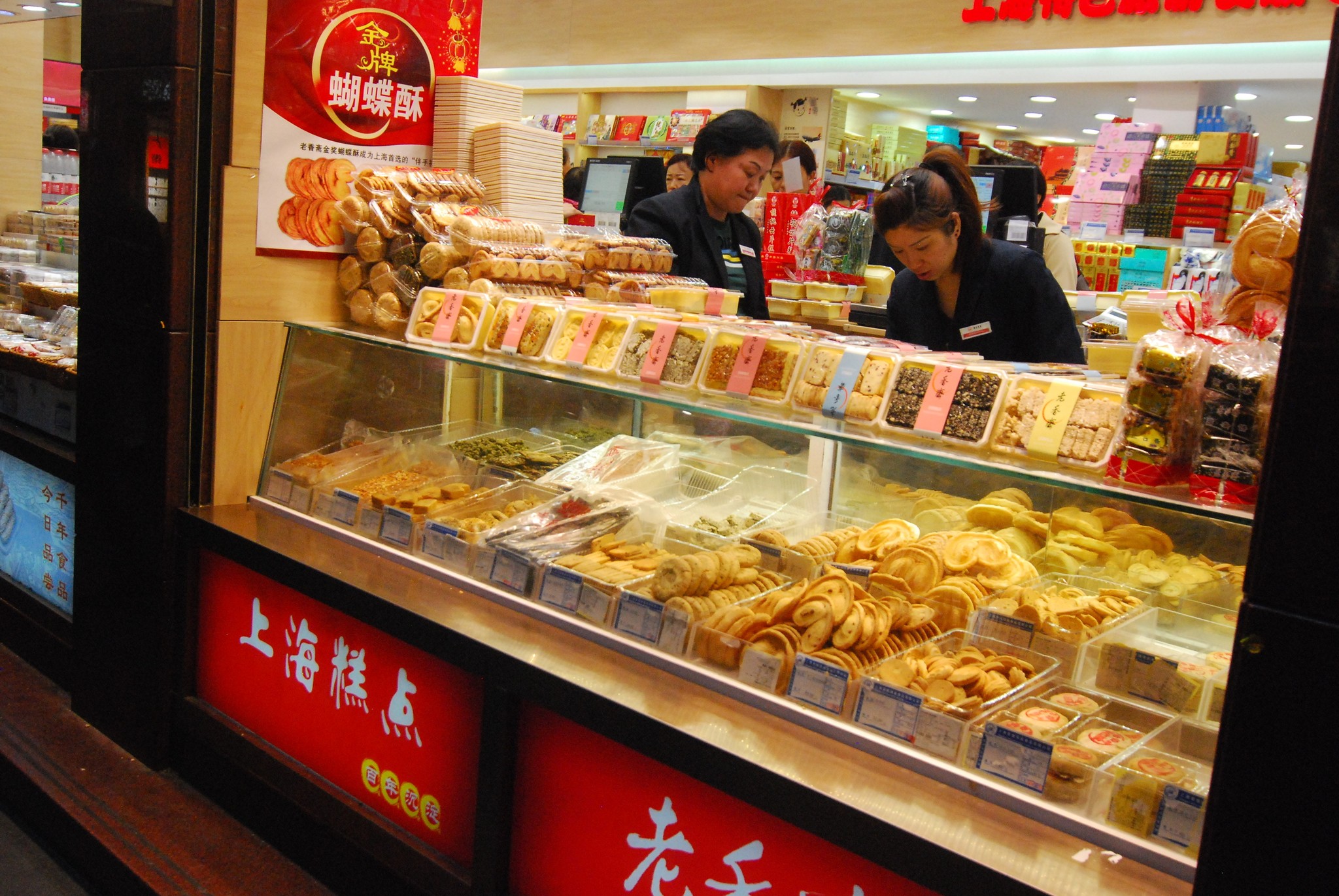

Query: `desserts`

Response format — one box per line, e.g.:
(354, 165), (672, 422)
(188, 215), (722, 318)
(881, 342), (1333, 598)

(991, 612), (1236, 857)
(410, 282), (1279, 487)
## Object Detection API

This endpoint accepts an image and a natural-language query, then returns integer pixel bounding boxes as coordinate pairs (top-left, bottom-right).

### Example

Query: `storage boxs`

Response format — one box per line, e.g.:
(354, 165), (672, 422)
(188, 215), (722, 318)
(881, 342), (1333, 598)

(273, 102), (1294, 860)
(0, 204), (79, 357)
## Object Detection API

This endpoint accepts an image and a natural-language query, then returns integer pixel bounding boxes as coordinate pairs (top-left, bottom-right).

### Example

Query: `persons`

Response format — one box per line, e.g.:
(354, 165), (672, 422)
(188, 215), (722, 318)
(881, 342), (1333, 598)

(623, 110), (781, 319)
(563, 148), (587, 203)
(868, 221), (906, 276)
(767, 141), (818, 194)
(872, 150), (1087, 365)
(821, 185), (850, 215)
(1002, 161), (1078, 296)
(665, 153), (696, 192)
(42, 124), (80, 151)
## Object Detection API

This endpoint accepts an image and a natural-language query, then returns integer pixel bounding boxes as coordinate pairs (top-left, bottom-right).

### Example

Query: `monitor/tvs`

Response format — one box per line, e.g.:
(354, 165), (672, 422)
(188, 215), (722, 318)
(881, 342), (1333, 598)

(578, 154), (666, 218)
(966, 164), (1039, 239)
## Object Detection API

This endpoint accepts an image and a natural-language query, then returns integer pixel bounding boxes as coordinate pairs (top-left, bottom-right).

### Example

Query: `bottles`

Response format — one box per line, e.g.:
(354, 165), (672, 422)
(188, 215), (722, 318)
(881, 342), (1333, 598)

(1193, 172), (1233, 188)
(43, 148), (80, 206)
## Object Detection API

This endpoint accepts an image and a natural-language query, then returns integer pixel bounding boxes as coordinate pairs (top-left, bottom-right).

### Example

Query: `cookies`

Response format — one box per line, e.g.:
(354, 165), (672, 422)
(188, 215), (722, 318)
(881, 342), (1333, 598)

(337, 165), (701, 331)
(1222, 209), (1298, 340)
(555, 487), (1248, 720)
